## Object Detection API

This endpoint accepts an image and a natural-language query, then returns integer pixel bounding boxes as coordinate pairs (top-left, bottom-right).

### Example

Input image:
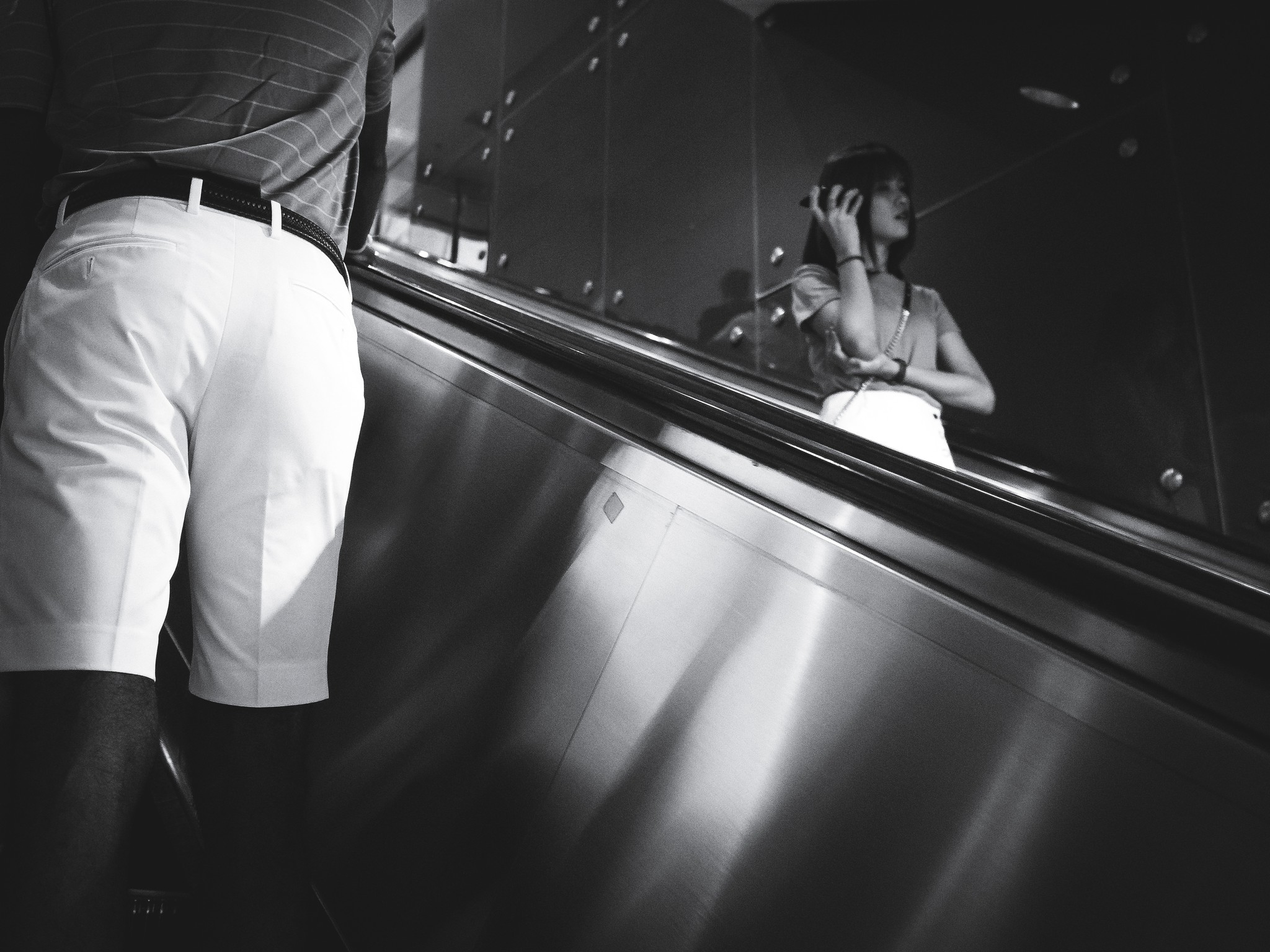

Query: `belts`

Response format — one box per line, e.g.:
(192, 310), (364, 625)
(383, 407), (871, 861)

(63, 166), (349, 293)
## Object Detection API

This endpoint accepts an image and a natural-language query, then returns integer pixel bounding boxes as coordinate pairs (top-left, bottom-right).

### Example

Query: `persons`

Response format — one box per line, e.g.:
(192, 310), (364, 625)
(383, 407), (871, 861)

(0, 0), (395, 952)
(790, 144), (997, 471)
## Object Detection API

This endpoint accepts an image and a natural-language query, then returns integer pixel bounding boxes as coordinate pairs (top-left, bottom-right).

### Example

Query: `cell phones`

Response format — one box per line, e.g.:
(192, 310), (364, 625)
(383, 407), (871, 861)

(798, 188), (866, 208)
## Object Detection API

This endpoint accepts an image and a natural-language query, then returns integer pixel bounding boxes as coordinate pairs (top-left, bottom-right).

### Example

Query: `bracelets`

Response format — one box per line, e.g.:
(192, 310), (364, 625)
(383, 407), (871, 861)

(836, 254), (866, 268)
(884, 357), (908, 386)
